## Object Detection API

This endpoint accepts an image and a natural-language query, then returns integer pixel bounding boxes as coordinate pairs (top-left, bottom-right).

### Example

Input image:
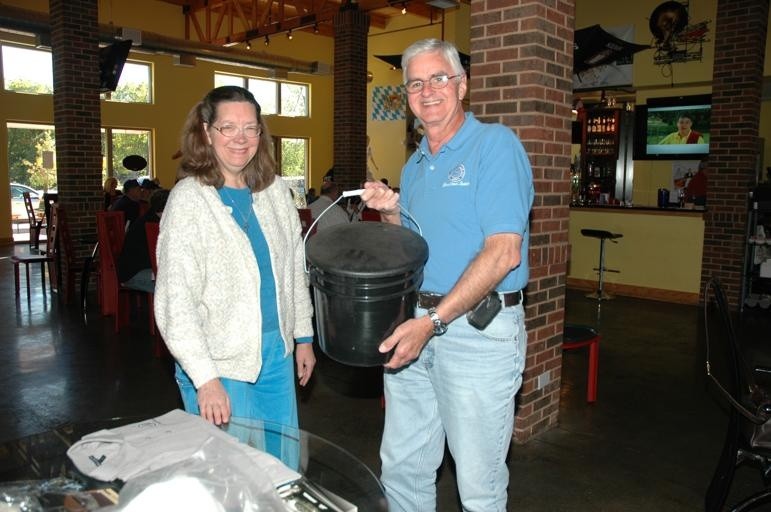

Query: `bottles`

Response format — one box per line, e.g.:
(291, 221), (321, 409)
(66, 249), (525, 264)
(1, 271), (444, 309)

(587, 184), (600, 204)
(584, 114), (615, 178)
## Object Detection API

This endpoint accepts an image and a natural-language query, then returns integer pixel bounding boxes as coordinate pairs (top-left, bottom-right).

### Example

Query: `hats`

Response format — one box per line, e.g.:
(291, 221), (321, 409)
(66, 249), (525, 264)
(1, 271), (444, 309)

(144, 179), (161, 189)
(124, 179), (144, 191)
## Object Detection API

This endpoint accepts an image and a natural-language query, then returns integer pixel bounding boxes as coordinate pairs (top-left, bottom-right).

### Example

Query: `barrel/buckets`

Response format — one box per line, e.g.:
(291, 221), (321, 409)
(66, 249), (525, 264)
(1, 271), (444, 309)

(300, 190), (431, 372)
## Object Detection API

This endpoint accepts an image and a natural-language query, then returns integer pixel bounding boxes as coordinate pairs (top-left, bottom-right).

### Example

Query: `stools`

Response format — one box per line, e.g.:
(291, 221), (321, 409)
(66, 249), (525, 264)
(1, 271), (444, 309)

(581, 229), (623, 301)
(562, 325), (601, 403)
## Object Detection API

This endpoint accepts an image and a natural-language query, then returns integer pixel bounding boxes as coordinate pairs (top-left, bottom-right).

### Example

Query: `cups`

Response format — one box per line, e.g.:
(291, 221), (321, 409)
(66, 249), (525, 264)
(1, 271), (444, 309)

(571, 192), (578, 205)
(578, 186), (586, 206)
(658, 188), (670, 207)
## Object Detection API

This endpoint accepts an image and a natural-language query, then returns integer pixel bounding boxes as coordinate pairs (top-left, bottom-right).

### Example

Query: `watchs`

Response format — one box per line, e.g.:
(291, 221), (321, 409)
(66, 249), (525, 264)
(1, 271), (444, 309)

(429, 305), (448, 336)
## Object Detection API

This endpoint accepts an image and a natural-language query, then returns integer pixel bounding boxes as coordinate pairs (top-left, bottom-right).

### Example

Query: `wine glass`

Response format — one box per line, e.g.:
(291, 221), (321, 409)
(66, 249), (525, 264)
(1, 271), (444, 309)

(678, 189), (686, 208)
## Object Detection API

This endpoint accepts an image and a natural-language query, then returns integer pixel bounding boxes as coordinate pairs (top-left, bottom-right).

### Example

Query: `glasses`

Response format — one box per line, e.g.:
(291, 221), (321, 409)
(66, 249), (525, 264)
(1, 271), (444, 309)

(204, 117), (260, 136)
(404, 73), (460, 95)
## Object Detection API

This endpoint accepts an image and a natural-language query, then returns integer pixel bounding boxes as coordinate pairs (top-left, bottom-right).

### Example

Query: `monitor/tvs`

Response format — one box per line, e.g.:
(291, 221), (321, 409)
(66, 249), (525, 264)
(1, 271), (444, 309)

(101, 40), (132, 90)
(633, 103), (711, 160)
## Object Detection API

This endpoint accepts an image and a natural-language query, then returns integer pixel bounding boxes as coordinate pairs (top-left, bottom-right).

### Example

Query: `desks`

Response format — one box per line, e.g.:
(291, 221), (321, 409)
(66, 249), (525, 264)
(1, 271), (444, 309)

(0, 415), (390, 512)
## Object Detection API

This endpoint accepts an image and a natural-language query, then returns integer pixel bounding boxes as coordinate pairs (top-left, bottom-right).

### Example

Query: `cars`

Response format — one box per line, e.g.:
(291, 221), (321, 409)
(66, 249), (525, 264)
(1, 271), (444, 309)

(9, 182), (48, 224)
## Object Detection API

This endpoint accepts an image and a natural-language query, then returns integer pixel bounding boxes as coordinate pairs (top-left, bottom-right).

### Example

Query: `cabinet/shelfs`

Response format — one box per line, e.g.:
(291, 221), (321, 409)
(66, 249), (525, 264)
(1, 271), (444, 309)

(741, 189), (771, 311)
(581, 109), (619, 194)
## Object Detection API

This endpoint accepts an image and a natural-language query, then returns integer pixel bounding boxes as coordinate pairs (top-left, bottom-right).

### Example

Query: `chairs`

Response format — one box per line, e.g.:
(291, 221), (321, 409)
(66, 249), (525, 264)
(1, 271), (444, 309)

(96, 211), (145, 333)
(10, 207), (58, 297)
(145, 222), (159, 336)
(23, 191), (48, 248)
(700, 276), (771, 511)
(299, 208), (314, 237)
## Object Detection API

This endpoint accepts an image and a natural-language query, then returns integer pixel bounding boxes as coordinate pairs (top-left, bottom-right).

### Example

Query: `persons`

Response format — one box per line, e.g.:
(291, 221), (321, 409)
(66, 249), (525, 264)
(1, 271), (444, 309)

(658, 114), (705, 144)
(152, 85), (317, 473)
(305, 168), (400, 232)
(358, 38), (534, 512)
(104, 176), (169, 284)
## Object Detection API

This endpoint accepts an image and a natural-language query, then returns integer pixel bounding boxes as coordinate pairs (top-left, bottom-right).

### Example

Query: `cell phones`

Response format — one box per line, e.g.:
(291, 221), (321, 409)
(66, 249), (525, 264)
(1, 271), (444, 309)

(468, 297), (501, 329)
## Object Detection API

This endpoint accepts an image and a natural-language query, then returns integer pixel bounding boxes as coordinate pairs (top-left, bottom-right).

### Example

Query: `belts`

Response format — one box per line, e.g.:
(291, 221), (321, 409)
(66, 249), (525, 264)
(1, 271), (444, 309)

(415, 290), (520, 307)
